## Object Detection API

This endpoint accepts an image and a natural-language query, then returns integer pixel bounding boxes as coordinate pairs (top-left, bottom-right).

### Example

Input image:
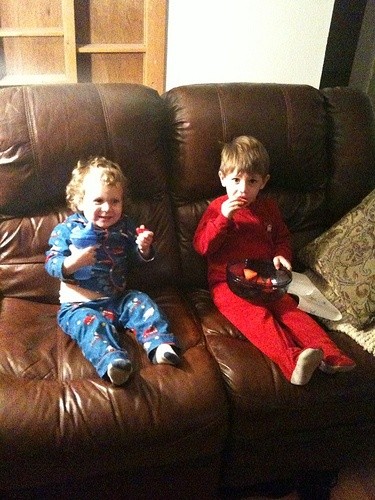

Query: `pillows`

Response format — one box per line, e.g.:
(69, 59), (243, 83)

(296, 190), (375, 332)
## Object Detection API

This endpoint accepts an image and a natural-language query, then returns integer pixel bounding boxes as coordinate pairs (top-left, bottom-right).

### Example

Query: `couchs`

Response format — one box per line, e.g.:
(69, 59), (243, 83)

(0, 83), (375, 499)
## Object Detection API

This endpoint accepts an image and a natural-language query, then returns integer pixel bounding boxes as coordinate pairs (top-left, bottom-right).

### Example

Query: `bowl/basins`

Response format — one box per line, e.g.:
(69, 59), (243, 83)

(225, 257), (292, 305)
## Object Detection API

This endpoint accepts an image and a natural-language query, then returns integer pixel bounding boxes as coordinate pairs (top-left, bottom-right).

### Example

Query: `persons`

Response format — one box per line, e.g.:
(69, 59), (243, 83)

(45, 155), (182, 387)
(193, 136), (356, 385)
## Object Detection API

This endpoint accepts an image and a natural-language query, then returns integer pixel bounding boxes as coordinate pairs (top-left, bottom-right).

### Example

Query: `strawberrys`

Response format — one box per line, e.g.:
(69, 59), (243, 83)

(135, 228), (147, 234)
(243, 268), (273, 292)
(238, 198), (249, 208)
